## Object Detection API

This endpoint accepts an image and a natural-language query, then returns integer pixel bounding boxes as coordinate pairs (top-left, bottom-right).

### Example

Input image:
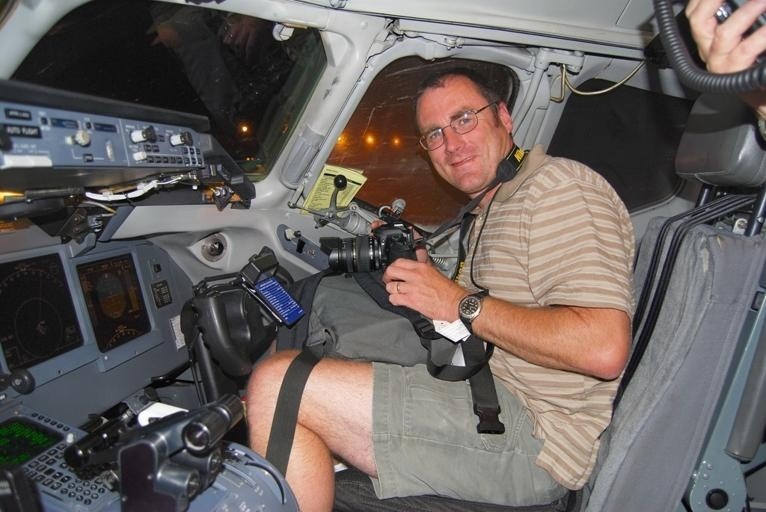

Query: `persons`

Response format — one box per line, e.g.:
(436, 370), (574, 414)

(680, 0), (766, 143)
(241, 68), (637, 510)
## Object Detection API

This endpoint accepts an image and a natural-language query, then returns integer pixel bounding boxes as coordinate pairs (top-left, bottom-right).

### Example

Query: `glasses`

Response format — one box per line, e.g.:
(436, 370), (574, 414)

(415, 100), (501, 152)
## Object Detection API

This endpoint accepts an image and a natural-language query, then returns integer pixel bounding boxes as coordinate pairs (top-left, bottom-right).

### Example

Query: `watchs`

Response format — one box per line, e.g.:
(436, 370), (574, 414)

(458, 287), (490, 337)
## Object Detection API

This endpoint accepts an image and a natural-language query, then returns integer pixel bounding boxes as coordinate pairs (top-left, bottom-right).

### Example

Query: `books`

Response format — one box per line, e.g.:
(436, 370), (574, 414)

(298, 163), (369, 215)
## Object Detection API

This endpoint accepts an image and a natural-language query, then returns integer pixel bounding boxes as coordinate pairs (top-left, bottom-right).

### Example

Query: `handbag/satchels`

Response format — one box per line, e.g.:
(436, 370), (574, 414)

(305, 268), (459, 367)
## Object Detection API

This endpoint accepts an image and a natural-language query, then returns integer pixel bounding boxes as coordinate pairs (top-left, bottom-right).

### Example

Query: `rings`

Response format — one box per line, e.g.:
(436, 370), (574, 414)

(396, 281), (402, 295)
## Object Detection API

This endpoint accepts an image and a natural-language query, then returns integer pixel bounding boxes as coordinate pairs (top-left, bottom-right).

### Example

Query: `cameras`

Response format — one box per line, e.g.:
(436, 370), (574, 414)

(320, 217), (418, 276)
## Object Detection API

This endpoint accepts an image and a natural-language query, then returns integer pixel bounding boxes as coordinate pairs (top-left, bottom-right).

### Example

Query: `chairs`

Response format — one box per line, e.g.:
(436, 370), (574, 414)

(570, 93), (765, 511)
